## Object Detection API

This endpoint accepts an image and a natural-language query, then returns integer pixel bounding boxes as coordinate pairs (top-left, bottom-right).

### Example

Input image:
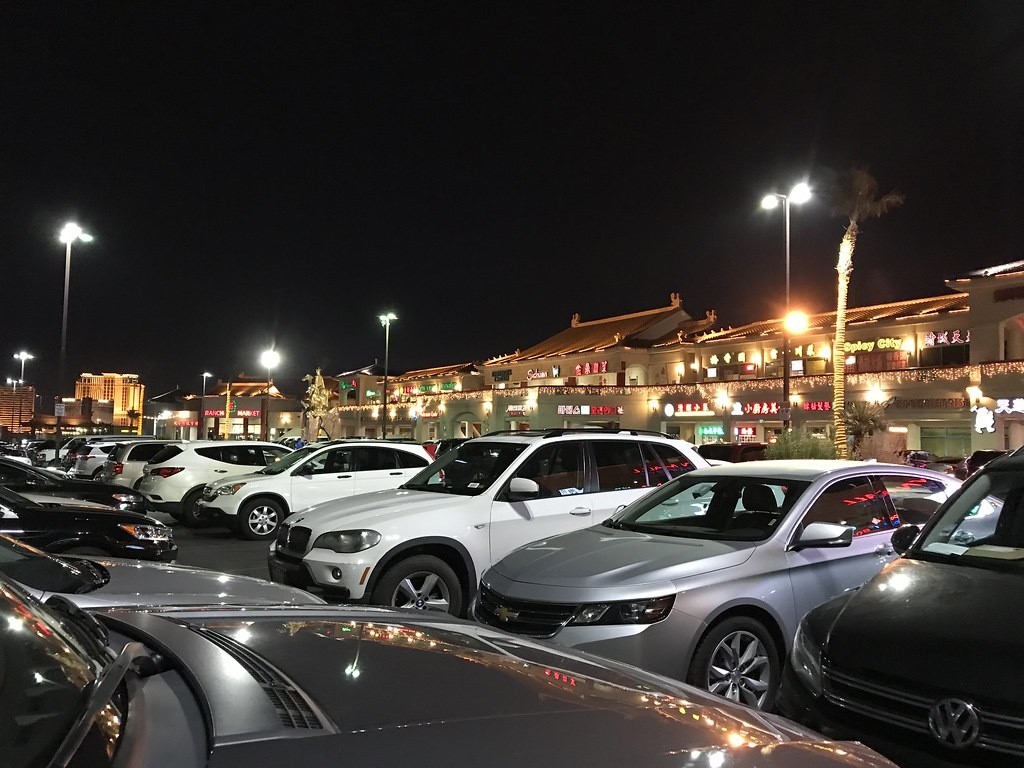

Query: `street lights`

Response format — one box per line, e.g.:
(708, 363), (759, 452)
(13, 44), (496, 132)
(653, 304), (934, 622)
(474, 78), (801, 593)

(6, 377), (23, 433)
(196, 371), (214, 440)
(760, 178), (815, 404)
(260, 349), (282, 438)
(376, 308), (399, 439)
(13, 351), (35, 432)
(781, 311), (809, 429)
(54, 222), (95, 461)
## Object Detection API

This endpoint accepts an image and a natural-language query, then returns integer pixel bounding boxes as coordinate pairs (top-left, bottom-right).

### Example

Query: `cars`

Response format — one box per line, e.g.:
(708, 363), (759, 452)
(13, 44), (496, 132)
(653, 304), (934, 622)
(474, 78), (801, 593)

(1, 457), (148, 515)
(762, 446), (1023, 767)
(894, 450), (938, 469)
(926, 455), (967, 481)
(1, 485), (178, 565)
(1, 571), (901, 767)
(468, 458), (1006, 714)
(0, 529), (329, 612)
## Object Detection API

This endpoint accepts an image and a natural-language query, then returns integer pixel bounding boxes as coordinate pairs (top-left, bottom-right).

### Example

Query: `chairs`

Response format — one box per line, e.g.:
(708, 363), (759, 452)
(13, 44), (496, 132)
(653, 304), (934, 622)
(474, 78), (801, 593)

(725, 484), (779, 541)
(333, 453), (347, 472)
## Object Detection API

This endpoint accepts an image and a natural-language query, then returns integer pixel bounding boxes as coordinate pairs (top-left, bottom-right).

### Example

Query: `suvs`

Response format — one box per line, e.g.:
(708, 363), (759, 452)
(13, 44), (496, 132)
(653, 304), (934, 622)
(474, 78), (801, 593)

(964, 450), (1015, 478)
(137, 438), (324, 527)
(1, 433), (155, 480)
(200, 436), (446, 541)
(267, 426), (787, 617)
(100, 440), (210, 494)
